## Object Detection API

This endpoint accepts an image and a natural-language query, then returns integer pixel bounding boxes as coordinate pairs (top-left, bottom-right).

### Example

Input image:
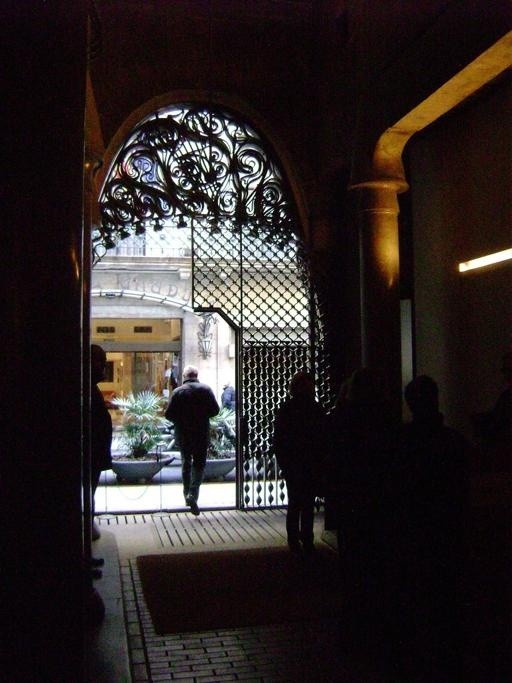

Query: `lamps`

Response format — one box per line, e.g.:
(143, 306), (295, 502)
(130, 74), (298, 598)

(197, 312), (218, 359)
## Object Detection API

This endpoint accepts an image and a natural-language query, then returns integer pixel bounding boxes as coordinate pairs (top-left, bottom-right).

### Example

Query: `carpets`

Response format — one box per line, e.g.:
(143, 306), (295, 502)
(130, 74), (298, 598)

(136, 540), (370, 636)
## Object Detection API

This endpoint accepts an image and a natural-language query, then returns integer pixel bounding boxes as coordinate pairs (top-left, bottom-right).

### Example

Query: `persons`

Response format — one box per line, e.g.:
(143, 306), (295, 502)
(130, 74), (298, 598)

(164, 364), (219, 514)
(382, 375), (476, 679)
(470, 349), (511, 473)
(90, 342), (112, 579)
(317, 380), (397, 658)
(274, 371), (327, 555)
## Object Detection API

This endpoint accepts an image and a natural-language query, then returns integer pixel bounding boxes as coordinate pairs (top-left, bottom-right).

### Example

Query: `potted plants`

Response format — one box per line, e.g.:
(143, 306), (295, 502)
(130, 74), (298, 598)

(180, 406), (236, 481)
(107, 390), (176, 481)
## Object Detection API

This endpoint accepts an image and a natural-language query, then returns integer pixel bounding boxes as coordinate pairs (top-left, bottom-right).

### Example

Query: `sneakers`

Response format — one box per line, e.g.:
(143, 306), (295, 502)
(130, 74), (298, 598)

(186, 492), (201, 516)
